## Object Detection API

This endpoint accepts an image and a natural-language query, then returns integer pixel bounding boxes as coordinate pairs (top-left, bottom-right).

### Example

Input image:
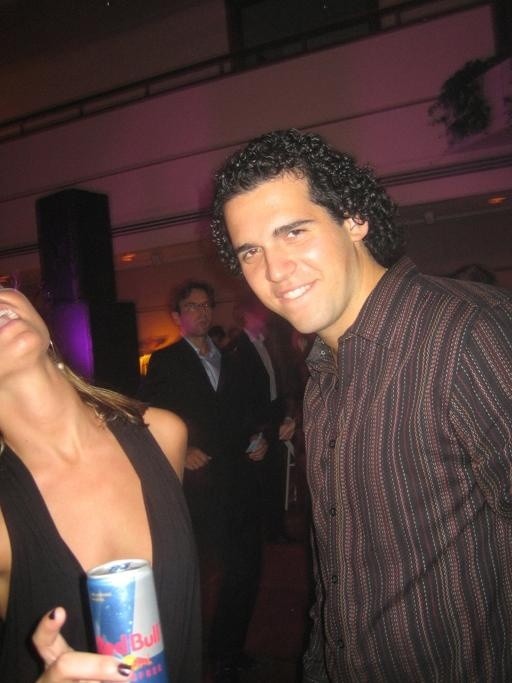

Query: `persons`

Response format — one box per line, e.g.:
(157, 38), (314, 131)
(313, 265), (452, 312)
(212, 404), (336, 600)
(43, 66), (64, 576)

(0, 276), (205, 683)
(141, 276), (312, 683)
(208, 137), (511, 683)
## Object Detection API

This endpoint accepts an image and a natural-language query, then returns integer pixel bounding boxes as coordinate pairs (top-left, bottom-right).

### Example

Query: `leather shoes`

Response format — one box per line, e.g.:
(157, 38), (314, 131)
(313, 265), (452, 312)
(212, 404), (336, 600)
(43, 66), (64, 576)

(263, 527), (297, 544)
(211, 654), (274, 678)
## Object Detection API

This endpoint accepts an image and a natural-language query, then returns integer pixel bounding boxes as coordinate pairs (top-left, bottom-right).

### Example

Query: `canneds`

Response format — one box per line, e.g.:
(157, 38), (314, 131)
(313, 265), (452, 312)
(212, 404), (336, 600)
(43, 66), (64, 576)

(85, 558), (169, 682)
